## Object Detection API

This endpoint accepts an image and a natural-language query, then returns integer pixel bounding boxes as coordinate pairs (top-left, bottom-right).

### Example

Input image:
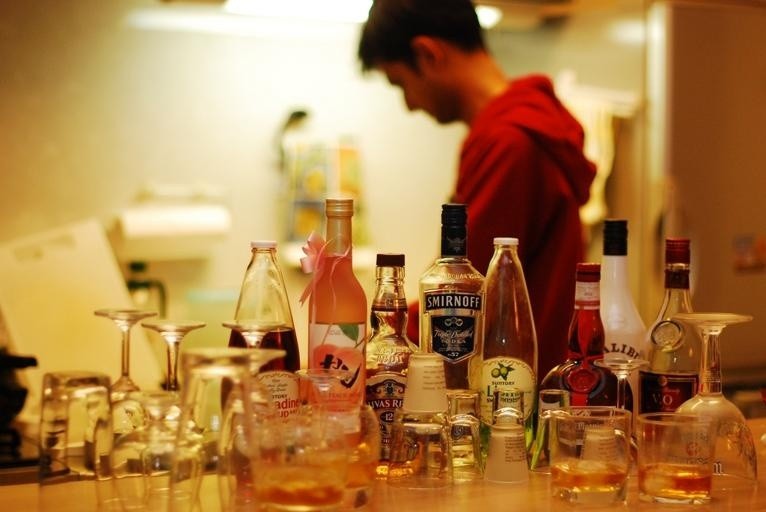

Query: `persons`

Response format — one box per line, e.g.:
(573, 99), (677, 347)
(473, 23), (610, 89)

(359, 0), (597, 390)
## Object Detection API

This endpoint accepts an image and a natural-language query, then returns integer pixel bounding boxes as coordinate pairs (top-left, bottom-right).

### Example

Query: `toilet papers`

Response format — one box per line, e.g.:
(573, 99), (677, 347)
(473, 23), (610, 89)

(115, 201), (233, 239)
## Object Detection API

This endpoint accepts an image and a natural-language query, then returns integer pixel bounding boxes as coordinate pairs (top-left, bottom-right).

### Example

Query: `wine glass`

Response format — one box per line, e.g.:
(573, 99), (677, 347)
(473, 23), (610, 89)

(667, 310), (761, 497)
(138, 317), (205, 512)
(92, 306), (158, 512)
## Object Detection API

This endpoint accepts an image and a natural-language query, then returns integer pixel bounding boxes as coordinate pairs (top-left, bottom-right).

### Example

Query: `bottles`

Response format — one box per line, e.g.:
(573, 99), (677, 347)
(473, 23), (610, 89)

(584, 215), (655, 440)
(632, 237), (707, 506)
(364, 250), (429, 478)
(474, 234), (542, 469)
(415, 202), (484, 406)
(306, 197), (370, 408)
(536, 261), (631, 467)
(218, 243), (303, 490)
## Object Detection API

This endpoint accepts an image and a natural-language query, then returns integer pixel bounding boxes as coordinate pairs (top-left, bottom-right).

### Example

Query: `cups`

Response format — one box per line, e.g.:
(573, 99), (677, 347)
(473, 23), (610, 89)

(92, 379), (212, 512)
(445, 390), (484, 483)
(166, 313), (388, 512)
(386, 407), (455, 488)
(543, 403), (630, 507)
(484, 405), (530, 484)
(628, 406), (716, 506)
(37, 366), (111, 482)
(401, 354), (449, 413)
